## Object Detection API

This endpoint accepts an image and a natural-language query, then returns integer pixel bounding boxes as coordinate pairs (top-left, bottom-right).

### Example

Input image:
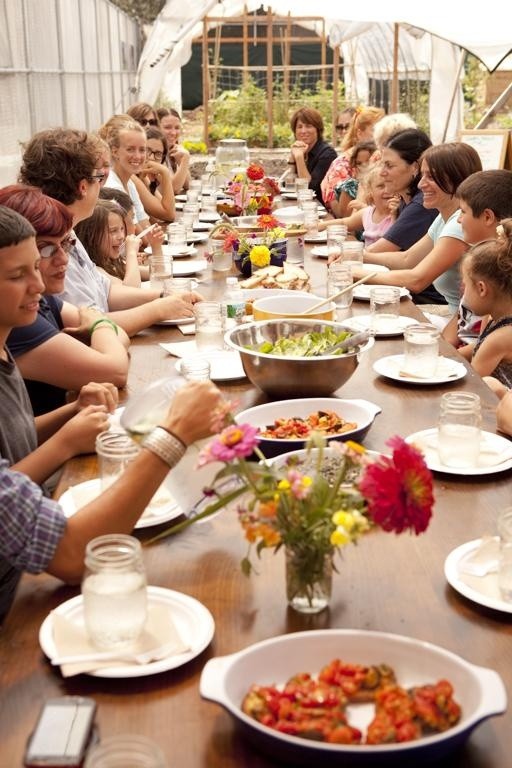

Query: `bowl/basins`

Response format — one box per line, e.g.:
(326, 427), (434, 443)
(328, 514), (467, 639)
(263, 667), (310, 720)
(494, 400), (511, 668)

(253, 294), (335, 322)
(233, 236), (289, 275)
(234, 398), (382, 449)
(216, 215), (265, 232)
(224, 318), (374, 398)
(199, 628), (507, 763)
(258, 447), (392, 502)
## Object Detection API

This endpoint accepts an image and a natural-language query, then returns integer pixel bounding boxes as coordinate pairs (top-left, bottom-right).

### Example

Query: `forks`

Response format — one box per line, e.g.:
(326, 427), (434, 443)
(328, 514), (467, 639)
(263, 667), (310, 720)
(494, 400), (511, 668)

(53, 644), (191, 668)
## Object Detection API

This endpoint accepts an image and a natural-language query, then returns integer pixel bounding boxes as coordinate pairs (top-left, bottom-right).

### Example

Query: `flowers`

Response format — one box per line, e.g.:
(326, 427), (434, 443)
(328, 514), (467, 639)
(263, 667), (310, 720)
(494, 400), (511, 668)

(212, 159), (281, 214)
(139, 396), (434, 568)
(204, 214), (313, 266)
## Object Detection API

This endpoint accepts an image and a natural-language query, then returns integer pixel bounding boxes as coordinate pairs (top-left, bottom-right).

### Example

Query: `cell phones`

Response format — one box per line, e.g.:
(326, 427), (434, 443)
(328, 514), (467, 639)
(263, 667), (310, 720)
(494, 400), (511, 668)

(24, 697), (98, 767)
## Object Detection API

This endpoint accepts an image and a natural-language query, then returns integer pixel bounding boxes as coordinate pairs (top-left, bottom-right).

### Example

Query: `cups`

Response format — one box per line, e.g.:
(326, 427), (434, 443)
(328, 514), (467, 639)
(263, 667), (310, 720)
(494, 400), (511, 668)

(439, 392), (481, 455)
(298, 188), (313, 208)
(303, 212), (318, 238)
(82, 534), (146, 647)
(403, 323), (439, 375)
(184, 202), (200, 227)
(327, 263), (353, 308)
(167, 223), (188, 251)
(498, 508), (512, 602)
(370, 287), (400, 329)
(188, 179), (202, 198)
(148, 256), (173, 290)
(174, 216), (194, 238)
(194, 301), (226, 348)
(186, 189), (198, 203)
(180, 358), (210, 383)
(211, 234), (233, 270)
(286, 234), (304, 263)
(294, 178), (308, 195)
(302, 202), (319, 217)
(327, 225), (347, 255)
(95, 431), (140, 493)
(285, 174), (295, 190)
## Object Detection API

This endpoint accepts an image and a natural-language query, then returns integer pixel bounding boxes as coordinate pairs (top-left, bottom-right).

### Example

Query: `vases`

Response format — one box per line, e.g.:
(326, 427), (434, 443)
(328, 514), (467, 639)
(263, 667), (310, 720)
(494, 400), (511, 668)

(285, 547), (333, 616)
(252, 194), (273, 214)
(235, 248), (284, 276)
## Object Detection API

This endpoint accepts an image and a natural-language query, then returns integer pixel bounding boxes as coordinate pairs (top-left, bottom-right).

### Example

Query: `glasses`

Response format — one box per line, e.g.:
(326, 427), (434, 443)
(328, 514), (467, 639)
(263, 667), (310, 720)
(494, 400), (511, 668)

(336, 124), (350, 130)
(84, 172), (105, 183)
(135, 119), (157, 126)
(37, 237), (78, 258)
(145, 147), (165, 161)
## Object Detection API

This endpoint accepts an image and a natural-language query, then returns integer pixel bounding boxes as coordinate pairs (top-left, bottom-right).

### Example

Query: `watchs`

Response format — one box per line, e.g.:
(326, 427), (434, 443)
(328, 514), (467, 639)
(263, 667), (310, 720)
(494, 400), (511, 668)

(287, 162), (296, 166)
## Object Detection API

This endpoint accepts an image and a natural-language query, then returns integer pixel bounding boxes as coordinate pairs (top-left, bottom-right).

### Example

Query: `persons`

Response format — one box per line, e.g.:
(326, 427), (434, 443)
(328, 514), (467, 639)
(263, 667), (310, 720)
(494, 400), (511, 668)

(334, 106), (355, 139)
(320, 106), (385, 218)
(2, 379), (232, 617)
(443, 168), (512, 352)
(282, 106), (339, 195)
(131, 127), (176, 222)
(333, 141), (376, 218)
(346, 114), (417, 215)
(327, 142), (482, 333)
(158, 106), (192, 197)
(74, 198), (168, 288)
(460, 220), (512, 403)
(0, 203), (120, 482)
(98, 188), (135, 237)
(98, 114), (167, 265)
(125, 103), (161, 132)
(364, 128), (449, 305)
(90, 133), (110, 188)
(317, 159), (401, 249)
(1, 185), (133, 417)
(19, 128), (203, 334)
(314, 166), (401, 252)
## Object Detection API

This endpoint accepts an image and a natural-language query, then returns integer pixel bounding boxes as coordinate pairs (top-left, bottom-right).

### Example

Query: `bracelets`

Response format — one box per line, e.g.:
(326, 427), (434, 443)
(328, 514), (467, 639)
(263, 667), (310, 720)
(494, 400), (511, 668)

(142, 426), (186, 469)
(159, 289), (165, 298)
(83, 318), (121, 333)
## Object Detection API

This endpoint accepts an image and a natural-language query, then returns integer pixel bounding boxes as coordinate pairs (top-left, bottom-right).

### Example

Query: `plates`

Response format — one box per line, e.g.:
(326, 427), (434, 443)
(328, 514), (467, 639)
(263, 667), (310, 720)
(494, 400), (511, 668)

(144, 244), (197, 257)
(304, 234), (327, 242)
(156, 317), (195, 325)
(403, 426), (512, 475)
(164, 233), (209, 243)
(199, 212), (221, 222)
(59, 474), (188, 529)
(174, 352), (246, 381)
(317, 210), (327, 218)
(444, 536), (512, 614)
(40, 585), (215, 678)
(281, 193), (317, 200)
(193, 223), (215, 231)
(341, 313), (419, 337)
(140, 278), (199, 291)
(351, 285), (410, 301)
(173, 261), (208, 277)
(175, 202), (202, 210)
(175, 194), (203, 201)
(316, 206), (325, 210)
(373, 353), (467, 385)
(106, 406), (127, 435)
(312, 240), (363, 260)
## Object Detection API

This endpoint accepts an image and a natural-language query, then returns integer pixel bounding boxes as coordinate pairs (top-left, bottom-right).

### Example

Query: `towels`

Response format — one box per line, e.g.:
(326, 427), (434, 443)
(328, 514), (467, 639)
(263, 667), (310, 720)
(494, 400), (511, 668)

(399, 355), (459, 378)
(431, 433), (511, 468)
(180, 243), (194, 254)
(51, 602), (192, 680)
(463, 537), (501, 575)
